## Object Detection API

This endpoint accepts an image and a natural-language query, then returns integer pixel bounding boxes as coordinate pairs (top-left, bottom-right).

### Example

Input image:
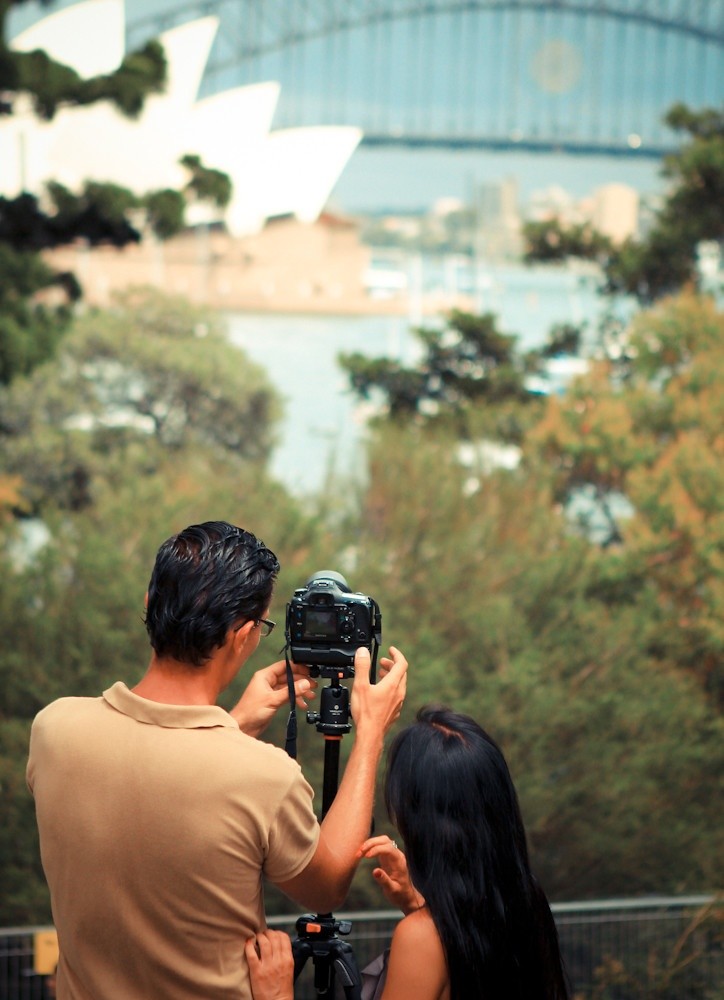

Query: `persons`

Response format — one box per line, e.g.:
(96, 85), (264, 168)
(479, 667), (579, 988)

(245, 706), (567, 1000)
(23, 521), (409, 1000)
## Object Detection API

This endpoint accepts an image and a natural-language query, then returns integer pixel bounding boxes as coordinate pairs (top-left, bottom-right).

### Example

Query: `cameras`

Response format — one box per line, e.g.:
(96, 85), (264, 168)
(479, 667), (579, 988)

(287, 569), (382, 678)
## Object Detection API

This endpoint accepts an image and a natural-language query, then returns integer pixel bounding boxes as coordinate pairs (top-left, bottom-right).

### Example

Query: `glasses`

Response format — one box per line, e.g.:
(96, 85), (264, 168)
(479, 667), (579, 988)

(231, 617), (277, 640)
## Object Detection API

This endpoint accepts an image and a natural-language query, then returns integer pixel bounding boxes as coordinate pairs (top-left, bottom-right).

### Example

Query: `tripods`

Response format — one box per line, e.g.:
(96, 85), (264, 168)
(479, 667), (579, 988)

(288, 677), (366, 1000)
